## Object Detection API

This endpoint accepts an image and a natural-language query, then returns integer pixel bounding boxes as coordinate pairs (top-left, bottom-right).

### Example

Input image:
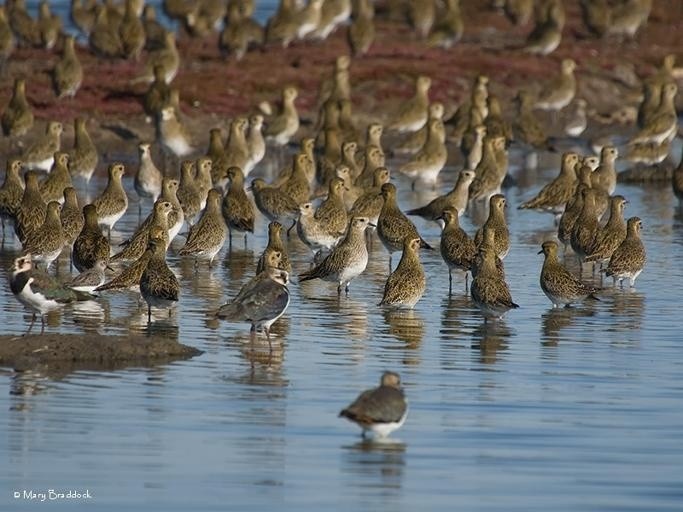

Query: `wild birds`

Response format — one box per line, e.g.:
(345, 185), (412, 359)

(0, 0), (683, 348)
(337, 369), (409, 439)
